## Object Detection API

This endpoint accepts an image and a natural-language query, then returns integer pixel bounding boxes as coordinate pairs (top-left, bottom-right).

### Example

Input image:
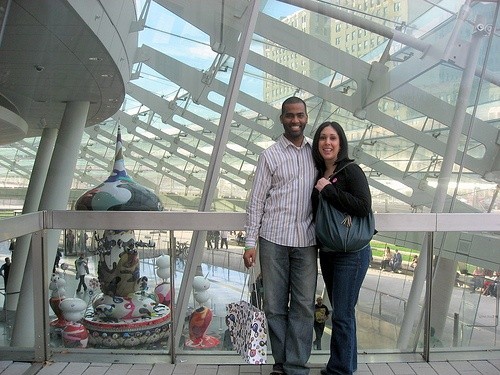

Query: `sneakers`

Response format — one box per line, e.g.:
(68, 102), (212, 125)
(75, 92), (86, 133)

(321, 369), (353, 375)
(271, 366), (282, 375)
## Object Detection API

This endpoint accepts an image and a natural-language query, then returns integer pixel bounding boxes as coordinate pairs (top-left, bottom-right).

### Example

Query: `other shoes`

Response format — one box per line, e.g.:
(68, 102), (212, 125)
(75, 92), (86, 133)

(313, 344), (317, 350)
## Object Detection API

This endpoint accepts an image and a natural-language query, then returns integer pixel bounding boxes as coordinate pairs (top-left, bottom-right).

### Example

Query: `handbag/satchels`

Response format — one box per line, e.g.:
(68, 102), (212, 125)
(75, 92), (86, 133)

(315, 163), (375, 253)
(226, 262), (267, 365)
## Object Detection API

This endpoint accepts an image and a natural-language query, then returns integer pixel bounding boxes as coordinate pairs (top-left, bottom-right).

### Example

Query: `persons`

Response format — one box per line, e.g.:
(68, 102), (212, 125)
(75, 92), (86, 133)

(65, 229), (90, 254)
(312, 297), (330, 352)
(206, 229), (229, 249)
(244, 96), (319, 375)
(312, 122), (378, 375)
(237, 231), (246, 248)
(471, 266), (499, 297)
(410, 255), (419, 275)
(74, 253), (91, 294)
(380, 246), (393, 270)
(391, 249), (403, 272)
(0, 256), (11, 293)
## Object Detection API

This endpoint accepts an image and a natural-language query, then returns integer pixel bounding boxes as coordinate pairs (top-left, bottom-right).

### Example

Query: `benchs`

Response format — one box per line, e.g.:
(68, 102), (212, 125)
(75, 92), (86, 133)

(370, 255), (485, 289)
